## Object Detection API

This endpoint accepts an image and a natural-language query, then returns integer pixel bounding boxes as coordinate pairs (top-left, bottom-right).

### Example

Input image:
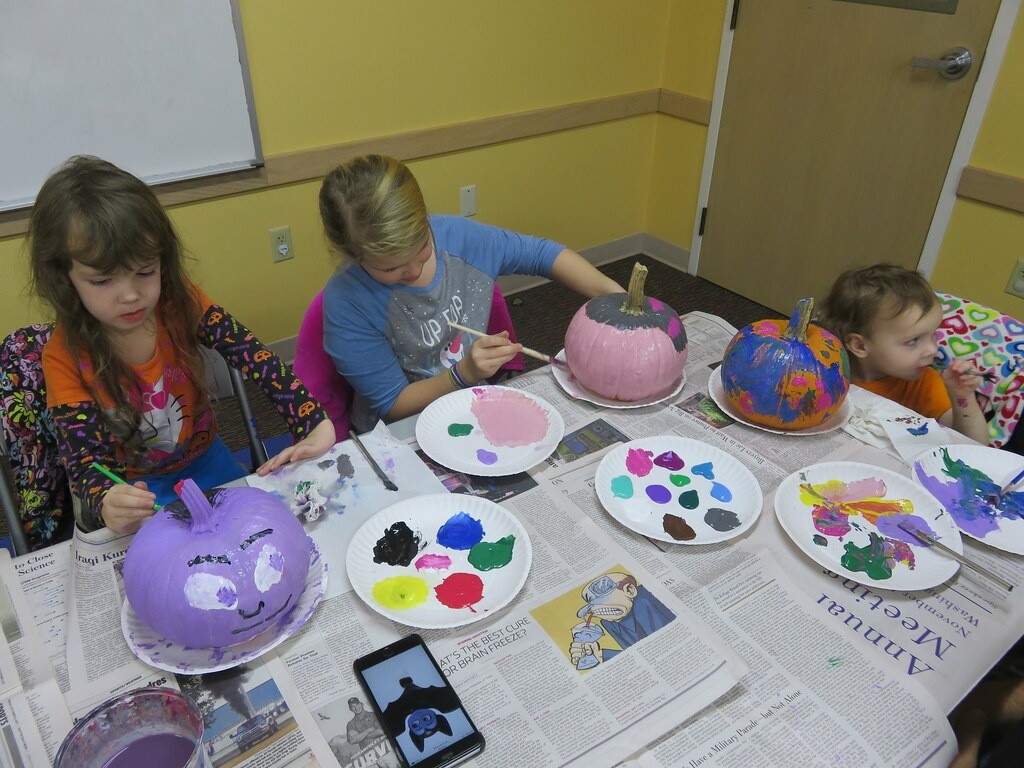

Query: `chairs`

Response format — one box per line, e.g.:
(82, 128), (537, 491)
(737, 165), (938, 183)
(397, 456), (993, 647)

(811, 289), (1024, 448)
(294, 280), (525, 445)
(0, 322), (274, 555)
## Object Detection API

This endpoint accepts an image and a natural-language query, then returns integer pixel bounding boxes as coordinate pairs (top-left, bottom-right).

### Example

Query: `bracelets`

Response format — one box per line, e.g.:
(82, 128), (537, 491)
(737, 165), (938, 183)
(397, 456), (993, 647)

(448, 362), (480, 390)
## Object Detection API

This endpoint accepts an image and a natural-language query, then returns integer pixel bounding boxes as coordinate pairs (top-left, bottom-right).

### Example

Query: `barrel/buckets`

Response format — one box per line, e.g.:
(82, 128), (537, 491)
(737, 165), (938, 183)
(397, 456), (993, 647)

(54, 686), (212, 768)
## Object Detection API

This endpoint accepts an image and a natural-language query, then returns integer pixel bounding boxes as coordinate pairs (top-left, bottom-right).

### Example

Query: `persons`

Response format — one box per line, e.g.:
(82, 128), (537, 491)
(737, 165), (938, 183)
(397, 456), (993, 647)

(20, 155), (336, 534)
(318, 154), (627, 435)
(209, 740), (215, 753)
(346, 697), (392, 763)
(816, 263), (989, 446)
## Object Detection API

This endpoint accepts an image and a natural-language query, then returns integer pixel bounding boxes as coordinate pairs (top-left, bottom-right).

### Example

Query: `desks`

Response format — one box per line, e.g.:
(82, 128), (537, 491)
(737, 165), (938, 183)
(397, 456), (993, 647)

(0, 309), (1024, 768)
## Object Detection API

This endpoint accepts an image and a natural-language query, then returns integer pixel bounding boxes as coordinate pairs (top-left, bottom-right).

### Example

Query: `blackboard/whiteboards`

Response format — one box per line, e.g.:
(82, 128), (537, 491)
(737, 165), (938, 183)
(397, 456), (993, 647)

(0, 0), (265, 213)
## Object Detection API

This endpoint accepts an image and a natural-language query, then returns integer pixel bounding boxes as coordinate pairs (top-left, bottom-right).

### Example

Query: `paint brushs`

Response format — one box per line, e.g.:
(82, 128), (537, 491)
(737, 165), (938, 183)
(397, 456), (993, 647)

(92, 461), (162, 511)
(1000, 470), (1024, 496)
(349, 430), (399, 491)
(930, 364), (996, 383)
(896, 519), (1014, 592)
(446, 321), (570, 373)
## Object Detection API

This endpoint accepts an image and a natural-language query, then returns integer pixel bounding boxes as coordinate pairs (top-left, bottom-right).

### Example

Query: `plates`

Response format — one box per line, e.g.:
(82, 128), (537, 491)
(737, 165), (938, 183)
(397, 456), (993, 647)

(912, 444), (1024, 556)
(774, 461), (963, 590)
(346, 493), (531, 628)
(415, 385), (564, 476)
(595, 436), (763, 546)
(120, 531), (327, 676)
(707, 365), (852, 435)
(551, 348), (686, 409)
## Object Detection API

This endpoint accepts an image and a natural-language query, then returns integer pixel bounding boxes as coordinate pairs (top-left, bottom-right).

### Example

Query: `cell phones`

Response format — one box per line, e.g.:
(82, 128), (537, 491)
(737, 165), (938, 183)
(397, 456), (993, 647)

(352, 634), (485, 768)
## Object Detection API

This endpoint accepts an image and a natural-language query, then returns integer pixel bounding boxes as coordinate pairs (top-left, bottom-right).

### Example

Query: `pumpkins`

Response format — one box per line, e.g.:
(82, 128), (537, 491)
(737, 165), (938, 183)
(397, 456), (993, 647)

(124, 479), (309, 645)
(565, 264), (689, 400)
(722, 297), (851, 428)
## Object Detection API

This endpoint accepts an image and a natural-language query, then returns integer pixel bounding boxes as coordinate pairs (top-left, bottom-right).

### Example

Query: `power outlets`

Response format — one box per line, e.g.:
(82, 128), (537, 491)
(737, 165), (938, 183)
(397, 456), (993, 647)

(268, 225), (294, 264)
